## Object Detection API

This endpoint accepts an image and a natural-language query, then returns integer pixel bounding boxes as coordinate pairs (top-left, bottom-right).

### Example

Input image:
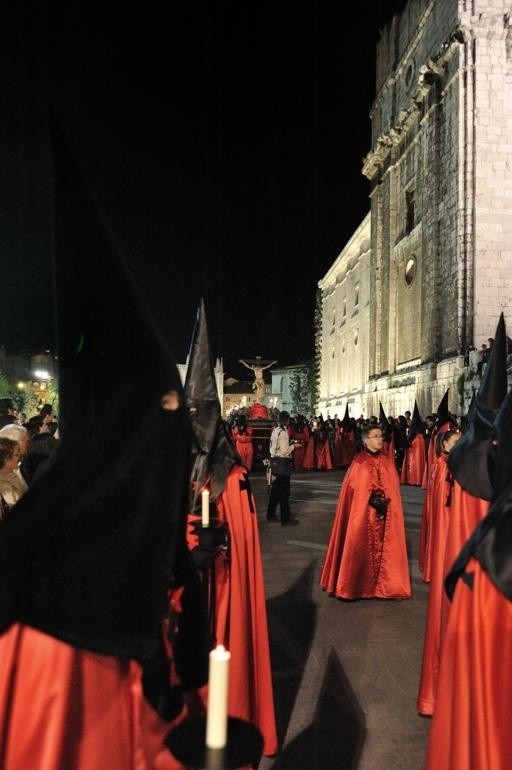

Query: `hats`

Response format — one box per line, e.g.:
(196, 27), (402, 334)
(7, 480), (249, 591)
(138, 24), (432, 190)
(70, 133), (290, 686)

(234, 414), (245, 427)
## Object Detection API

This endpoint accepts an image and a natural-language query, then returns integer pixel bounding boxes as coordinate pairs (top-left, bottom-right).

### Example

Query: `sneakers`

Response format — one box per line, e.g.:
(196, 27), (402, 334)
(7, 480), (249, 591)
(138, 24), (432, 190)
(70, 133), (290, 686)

(282, 519), (299, 526)
(267, 514), (280, 522)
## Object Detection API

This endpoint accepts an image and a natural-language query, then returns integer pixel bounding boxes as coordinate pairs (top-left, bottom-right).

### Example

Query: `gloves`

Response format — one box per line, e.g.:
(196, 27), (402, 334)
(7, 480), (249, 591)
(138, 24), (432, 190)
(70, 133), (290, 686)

(369, 494), (390, 514)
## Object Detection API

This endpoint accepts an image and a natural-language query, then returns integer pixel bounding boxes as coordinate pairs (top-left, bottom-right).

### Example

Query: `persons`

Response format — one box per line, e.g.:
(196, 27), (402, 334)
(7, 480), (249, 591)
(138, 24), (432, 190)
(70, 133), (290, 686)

(2, 310), (512, 769)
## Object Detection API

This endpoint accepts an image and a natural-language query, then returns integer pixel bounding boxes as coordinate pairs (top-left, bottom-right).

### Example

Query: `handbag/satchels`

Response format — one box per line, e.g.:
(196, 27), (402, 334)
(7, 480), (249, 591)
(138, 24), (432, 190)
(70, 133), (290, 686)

(269, 460), (292, 476)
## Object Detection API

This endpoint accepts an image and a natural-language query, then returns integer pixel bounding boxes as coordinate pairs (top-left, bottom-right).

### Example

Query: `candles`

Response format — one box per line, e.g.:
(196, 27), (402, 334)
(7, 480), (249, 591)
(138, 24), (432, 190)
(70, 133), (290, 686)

(201, 488), (210, 525)
(206, 644), (231, 750)
